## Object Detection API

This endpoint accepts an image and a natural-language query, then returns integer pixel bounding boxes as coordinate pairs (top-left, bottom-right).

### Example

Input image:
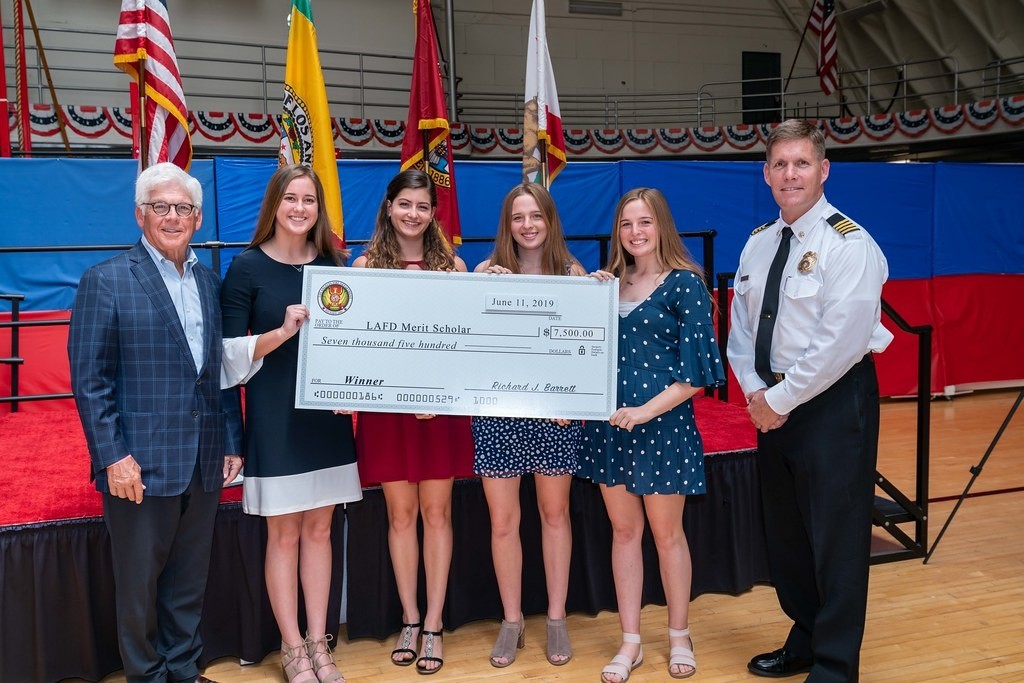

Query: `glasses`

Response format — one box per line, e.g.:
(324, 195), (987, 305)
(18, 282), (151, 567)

(143, 201), (198, 218)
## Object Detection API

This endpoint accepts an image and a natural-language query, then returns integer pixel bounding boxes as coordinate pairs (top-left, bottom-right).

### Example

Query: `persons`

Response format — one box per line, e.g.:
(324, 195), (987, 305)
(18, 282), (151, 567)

(470, 183), (726, 683)
(220, 164), (363, 683)
(350, 169), (478, 683)
(726, 119), (894, 683)
(68, 162), (246, 683)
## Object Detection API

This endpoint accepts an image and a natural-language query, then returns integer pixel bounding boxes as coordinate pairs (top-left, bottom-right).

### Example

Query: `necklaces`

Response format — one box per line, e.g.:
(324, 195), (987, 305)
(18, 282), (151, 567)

(271, 243), (304, 273)
(625, 278), (643, 285)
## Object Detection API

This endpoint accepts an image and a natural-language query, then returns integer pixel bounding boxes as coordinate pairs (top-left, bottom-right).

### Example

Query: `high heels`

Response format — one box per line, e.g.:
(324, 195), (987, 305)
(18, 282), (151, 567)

(490, 612), (526, 668)
(280, 630), (347, 683)
(391, 622), (443, 674)
(546, 615), (572, 666)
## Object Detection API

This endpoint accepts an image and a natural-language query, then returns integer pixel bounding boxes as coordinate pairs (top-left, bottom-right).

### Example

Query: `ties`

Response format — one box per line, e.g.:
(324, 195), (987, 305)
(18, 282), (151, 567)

(755, 227), (793, 387)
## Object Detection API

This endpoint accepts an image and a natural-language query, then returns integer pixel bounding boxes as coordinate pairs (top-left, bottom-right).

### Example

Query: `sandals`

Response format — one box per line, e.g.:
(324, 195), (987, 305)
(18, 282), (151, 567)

(668, 626), (697, 679)
(601, 632), (644, 683)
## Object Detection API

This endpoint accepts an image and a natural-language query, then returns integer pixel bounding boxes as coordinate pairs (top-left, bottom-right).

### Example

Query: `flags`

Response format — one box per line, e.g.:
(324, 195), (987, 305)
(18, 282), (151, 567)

(522, 0), (567, 191)
(279, 0), (347, 259)
(113, 0), (193, 172)
(399, 0), (464, 252)
(808, 0), (841, 96)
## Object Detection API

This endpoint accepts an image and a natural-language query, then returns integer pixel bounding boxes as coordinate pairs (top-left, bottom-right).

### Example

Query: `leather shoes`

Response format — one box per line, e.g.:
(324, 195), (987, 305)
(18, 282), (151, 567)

(195, 676), (219, 683)
(747, 646), (811, 678)
